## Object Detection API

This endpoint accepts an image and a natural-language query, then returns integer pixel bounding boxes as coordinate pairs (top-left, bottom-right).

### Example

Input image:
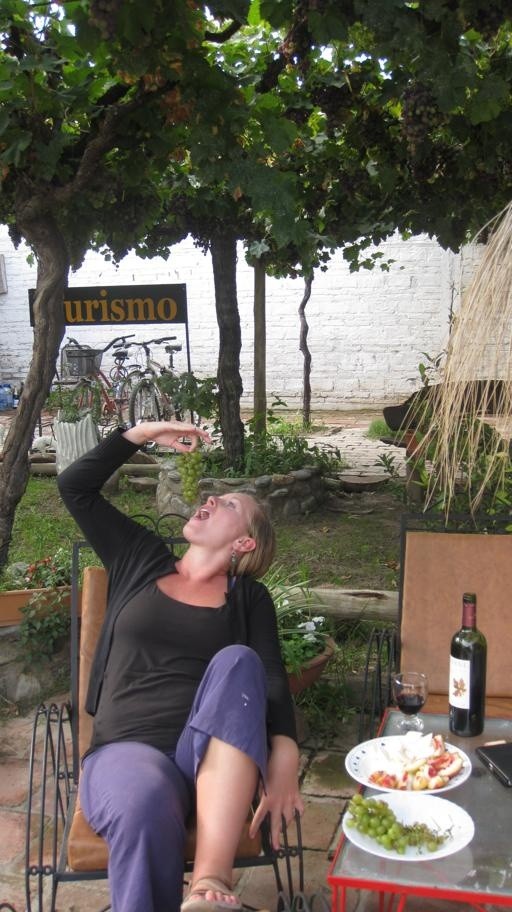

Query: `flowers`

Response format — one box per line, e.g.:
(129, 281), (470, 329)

(0, 548), (104, 674)
(255, 560), (330, 683)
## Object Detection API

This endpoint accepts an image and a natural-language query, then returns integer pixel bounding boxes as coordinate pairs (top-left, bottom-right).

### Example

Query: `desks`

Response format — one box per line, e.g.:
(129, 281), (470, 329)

(325, 706), (512, 912)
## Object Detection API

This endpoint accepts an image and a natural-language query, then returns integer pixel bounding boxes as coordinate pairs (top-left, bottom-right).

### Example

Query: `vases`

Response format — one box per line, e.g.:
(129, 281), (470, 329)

(0, 584), (83, 627)
(285, 634), (335, 696)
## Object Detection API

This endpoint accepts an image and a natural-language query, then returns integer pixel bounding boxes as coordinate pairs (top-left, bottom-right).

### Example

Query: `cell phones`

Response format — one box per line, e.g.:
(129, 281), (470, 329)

(475, 739), (512, 788)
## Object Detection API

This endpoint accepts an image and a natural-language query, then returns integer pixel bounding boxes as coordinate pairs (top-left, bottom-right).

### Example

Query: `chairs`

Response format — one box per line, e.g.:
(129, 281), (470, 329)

(358, 491), (512, 743)
(0, 513), (331, 912)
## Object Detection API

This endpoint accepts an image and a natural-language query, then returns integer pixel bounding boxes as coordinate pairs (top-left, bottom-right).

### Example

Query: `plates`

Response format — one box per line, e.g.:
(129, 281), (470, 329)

(341, 792), (477, 864)
(344, 733), (474, 794)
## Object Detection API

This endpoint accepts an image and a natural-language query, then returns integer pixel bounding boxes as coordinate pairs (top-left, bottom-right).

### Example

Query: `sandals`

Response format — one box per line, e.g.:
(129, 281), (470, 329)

(180, 876), (243, 912)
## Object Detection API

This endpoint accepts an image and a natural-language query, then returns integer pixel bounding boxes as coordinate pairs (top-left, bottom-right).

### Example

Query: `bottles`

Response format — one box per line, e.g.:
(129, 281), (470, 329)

(447, 593), (488, 738)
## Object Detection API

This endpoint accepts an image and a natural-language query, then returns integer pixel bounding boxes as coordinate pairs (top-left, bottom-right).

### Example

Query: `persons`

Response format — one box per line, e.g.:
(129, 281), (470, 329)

(55, 420), (304, 912)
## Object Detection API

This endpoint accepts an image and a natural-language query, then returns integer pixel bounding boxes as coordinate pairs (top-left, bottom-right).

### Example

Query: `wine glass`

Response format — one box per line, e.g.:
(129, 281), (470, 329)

(390, 671), (430, 733)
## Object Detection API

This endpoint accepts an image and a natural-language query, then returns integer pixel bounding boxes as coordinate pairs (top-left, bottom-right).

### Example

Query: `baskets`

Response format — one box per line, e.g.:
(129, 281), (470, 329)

(66, 350), (102, 376)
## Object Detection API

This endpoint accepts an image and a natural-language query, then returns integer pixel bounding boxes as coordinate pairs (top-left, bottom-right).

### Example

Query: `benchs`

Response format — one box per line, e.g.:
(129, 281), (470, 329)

(380, 380), (512, 467)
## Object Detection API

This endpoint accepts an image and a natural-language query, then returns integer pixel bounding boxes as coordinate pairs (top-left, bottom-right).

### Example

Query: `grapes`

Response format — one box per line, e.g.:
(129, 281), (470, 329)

(174, 449), (206, 505)
(1, 0), (511, 267)
(346, 793), (438, 854)
